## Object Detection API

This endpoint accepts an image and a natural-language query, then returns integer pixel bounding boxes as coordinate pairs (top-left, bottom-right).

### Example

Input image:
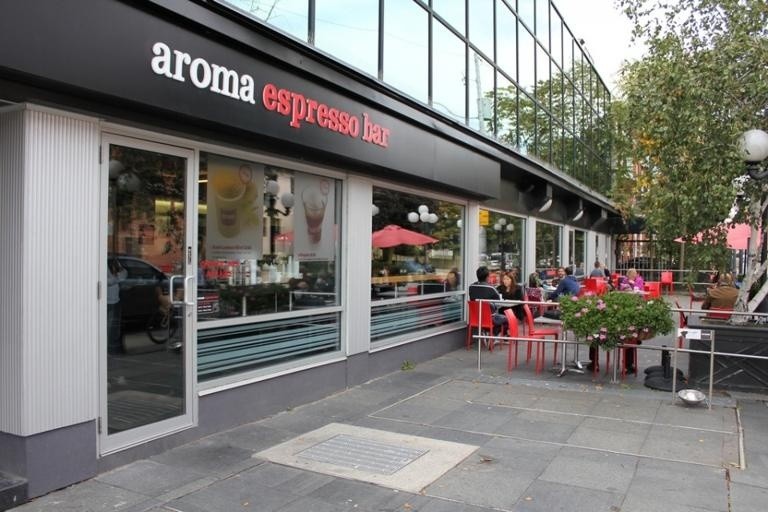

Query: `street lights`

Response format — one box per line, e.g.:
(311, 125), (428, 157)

(737, 188), (768, 286)
(457, 220), (462, 228)
(493, 218), (514, 271)
(264, 181), (294, 259)
(407, 202), (437, 272)
(372, 205), (380, 216)
(735, 128), (768, 183)
(109, 160), (139, 258)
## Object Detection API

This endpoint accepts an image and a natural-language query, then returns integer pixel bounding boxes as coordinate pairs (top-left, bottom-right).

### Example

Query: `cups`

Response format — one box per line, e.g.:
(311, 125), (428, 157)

(634, 286), (639, 293)
(301, 184), (328, 243)
(210, 170), (248, 238)
(206, 257), (301, 285)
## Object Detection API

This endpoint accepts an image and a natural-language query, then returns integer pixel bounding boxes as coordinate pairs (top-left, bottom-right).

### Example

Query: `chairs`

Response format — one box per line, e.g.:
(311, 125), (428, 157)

(708, 307), (734, 319)
(686, 283), (708, 315)
(406, 264), (674, 376)
(675, 299), (688, 348)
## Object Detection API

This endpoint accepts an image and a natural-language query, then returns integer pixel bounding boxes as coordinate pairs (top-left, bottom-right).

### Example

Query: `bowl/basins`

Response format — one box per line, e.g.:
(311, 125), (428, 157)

(678, 388), (705, 405)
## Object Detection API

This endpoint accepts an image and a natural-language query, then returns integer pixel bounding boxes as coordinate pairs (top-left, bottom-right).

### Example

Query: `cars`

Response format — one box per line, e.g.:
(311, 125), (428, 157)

(620, 257), (672, 280)
(108, 257), (183, 331)
(371, 258), (425, 286)
(480, 253), (561, 270)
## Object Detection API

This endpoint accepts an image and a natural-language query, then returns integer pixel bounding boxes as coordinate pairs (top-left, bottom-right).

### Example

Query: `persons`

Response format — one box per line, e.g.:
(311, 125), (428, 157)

(404, 256), (425, 275)
(701, 270), (741, 311)
(469, 262), (648, 378)
(441, 266), (461, 290)
(386, 258), (400, 274)
(108, 255), (129, 355)
(422, 246), (437, 272)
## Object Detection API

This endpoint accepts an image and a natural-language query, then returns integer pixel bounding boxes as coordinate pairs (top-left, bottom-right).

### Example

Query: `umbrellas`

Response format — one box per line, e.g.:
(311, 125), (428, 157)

(372, 222), (441, 254)
(670, 216), (767, 251)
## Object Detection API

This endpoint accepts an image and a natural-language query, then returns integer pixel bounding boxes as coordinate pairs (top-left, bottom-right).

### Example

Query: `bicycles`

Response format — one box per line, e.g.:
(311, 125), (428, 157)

(145, 308), (182, 344)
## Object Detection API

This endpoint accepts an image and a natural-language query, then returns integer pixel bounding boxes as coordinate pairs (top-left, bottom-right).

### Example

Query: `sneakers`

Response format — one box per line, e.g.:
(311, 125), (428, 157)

(483, 330), (489, 346)
